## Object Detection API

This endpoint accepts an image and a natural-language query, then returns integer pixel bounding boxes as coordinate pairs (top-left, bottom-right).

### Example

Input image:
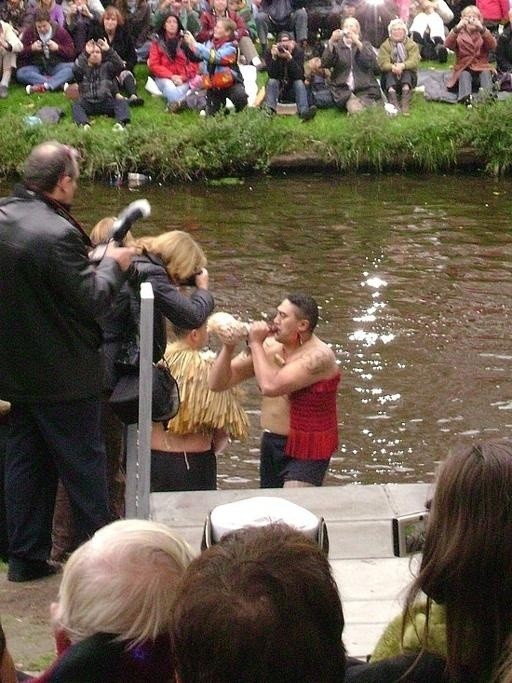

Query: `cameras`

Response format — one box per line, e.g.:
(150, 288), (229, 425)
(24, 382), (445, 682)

(179, 270), (201, 288)
(392, 510), (435, 560)
(278, 42), (288, 51)
(338, 29), (350, 36)
(39, 41), (48, 46)
(467, 17), (475, 24)
(179, 29), (188, 36)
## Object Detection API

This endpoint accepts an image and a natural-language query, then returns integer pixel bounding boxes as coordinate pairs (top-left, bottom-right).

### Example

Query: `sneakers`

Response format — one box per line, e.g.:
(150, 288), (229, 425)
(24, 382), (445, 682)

(129, 99), (144, 106)
(26, 85), (46, 95)
(0, 86), (8, 98)
(8, 558), (57, 581)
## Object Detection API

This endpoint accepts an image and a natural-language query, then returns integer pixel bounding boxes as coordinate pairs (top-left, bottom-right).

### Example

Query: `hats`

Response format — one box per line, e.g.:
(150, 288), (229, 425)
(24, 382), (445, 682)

(200, 494), (329, 560)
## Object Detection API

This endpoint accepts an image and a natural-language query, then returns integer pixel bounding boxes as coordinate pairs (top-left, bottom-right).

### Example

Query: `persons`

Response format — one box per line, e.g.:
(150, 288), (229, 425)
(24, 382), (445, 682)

(46, 229), (215, 563)
(1, 1), (511, 133)
(165, 520), (474, 683)
(369, 437), (512, 683)
(208, 295), (340, 489)
(17, 518), (196, 683)
(81, 214), (134, 267)
(147, 314), (249, 493)
(1, 142), (135, 583)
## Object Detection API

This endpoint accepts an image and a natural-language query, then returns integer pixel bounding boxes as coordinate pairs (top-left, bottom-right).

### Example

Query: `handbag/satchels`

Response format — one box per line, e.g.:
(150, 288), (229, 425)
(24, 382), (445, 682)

(313, 90), (335, 108)
(109, 365), (180, 423)
